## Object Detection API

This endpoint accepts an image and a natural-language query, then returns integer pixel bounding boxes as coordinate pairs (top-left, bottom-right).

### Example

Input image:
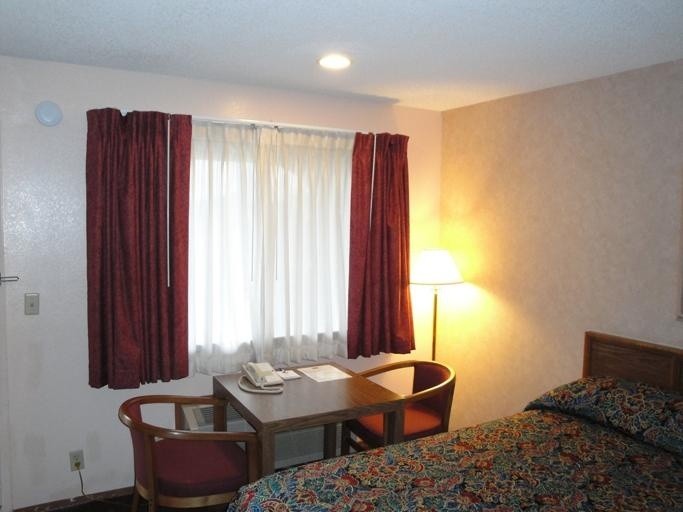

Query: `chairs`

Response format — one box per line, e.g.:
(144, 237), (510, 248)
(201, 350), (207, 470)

(341, 360), (457, 456)
(117, 394), (258, 511)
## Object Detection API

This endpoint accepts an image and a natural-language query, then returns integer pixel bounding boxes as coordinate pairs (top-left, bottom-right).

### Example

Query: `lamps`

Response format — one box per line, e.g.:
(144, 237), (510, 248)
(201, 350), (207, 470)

(406, 247), (468, 363)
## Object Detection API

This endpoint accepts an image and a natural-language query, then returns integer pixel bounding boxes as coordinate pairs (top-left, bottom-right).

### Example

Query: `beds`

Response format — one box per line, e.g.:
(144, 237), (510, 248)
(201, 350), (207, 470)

(226, 331), (682, 511)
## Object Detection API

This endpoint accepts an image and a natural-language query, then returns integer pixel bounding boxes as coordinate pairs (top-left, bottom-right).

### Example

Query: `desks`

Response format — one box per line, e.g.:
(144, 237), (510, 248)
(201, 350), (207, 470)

(212, 362), (407, 480)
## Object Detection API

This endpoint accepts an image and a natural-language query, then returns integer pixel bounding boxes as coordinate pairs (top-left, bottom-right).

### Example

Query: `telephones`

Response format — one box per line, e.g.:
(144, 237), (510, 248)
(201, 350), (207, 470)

(240, 362), (286, 387)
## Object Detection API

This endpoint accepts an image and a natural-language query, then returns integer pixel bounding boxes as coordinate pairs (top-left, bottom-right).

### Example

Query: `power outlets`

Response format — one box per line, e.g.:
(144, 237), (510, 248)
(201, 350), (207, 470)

(68, 448), (85, 473)
